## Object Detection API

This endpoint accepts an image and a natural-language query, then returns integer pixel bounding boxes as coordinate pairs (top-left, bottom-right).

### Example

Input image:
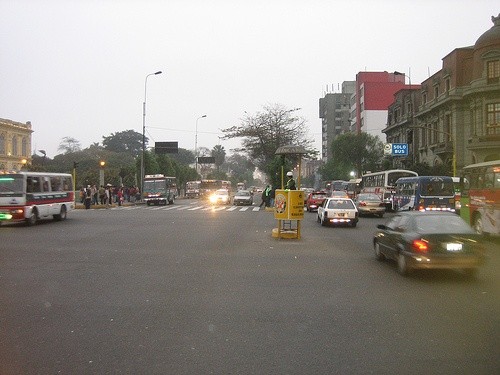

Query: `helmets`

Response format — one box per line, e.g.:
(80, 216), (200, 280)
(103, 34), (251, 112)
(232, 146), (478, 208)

(287, 172), (292, 176)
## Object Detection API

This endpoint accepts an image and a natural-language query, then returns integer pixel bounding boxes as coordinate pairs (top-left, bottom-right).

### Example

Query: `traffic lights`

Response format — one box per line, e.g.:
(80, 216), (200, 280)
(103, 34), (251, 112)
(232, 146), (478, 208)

(74, 161), (79, 168)
(100, 161), (106, 166)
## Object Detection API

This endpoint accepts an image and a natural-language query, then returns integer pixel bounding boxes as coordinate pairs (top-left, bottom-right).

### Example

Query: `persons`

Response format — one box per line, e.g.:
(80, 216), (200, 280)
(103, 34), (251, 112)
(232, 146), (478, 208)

(260, 185), (275, 208)
(285, 172), (296, 190)
(80, 183), (140, 206)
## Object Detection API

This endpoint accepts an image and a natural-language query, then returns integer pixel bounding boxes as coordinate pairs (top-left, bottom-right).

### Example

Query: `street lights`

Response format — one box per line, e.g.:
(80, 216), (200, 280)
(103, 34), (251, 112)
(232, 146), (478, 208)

(194, 115), (207, 171)
(142, 71), (162, 192)
(394, 71), (414, 171)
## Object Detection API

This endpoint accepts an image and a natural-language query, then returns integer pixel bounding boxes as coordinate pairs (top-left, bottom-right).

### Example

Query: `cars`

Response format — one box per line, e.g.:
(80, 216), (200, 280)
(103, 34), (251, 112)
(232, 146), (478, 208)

(317, 197), (359, 227)
(330, 191), (350, 198)
(214, 189), (231, 205)
(306, 191), (327, 212)
(353, 193), (386, 217)
(373, 211), (485, 277)
(233, 190), (253, 205)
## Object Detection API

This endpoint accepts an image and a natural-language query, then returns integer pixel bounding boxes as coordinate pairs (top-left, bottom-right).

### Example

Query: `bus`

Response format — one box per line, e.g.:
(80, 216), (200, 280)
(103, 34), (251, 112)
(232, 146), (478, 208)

(391, 176), (455, 212)
(451, 177), (470, 214)
(143, 174), (178, 206)
(319, 179), (362, 199)
(184, 180), (232, 199)
(459, 160), (500, 242)
(0, 172), (75, 226)
(360, 170), (419, 212)
